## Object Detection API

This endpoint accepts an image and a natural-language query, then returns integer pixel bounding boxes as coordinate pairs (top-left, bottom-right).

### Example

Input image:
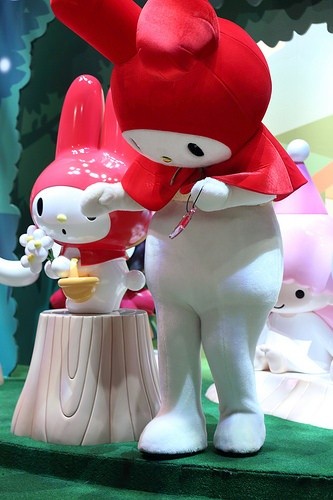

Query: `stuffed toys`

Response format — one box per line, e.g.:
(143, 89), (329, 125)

(51, 0), (309, 457)
(253, 139), (332, 374)
